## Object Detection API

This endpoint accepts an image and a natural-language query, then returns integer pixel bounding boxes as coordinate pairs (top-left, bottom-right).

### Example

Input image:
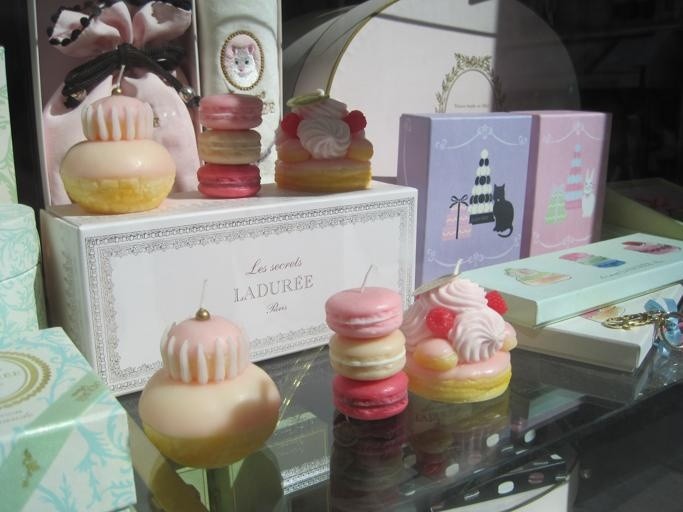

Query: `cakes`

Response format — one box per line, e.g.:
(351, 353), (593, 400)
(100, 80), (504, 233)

(274, 89), (374, 192)
(58, 87), (175, 215)
(138, 308), (282, 468)
(468, 148), (494, 214)
(400, 272), (518, 403)
(146, 444), (283, 512)
(563, 144), (584, 202)
(411, 388), (511, 479)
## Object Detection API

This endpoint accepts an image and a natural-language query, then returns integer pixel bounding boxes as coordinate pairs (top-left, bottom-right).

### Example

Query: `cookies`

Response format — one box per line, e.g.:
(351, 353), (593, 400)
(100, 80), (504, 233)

(324, 287), (410, 421)
(330, 414), (410, 512)
(195, 95), (263, 198)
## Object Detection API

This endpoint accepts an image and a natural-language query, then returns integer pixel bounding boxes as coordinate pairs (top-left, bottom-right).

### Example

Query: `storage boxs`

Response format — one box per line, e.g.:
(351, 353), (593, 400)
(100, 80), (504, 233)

(38, 176), (418, 398)
